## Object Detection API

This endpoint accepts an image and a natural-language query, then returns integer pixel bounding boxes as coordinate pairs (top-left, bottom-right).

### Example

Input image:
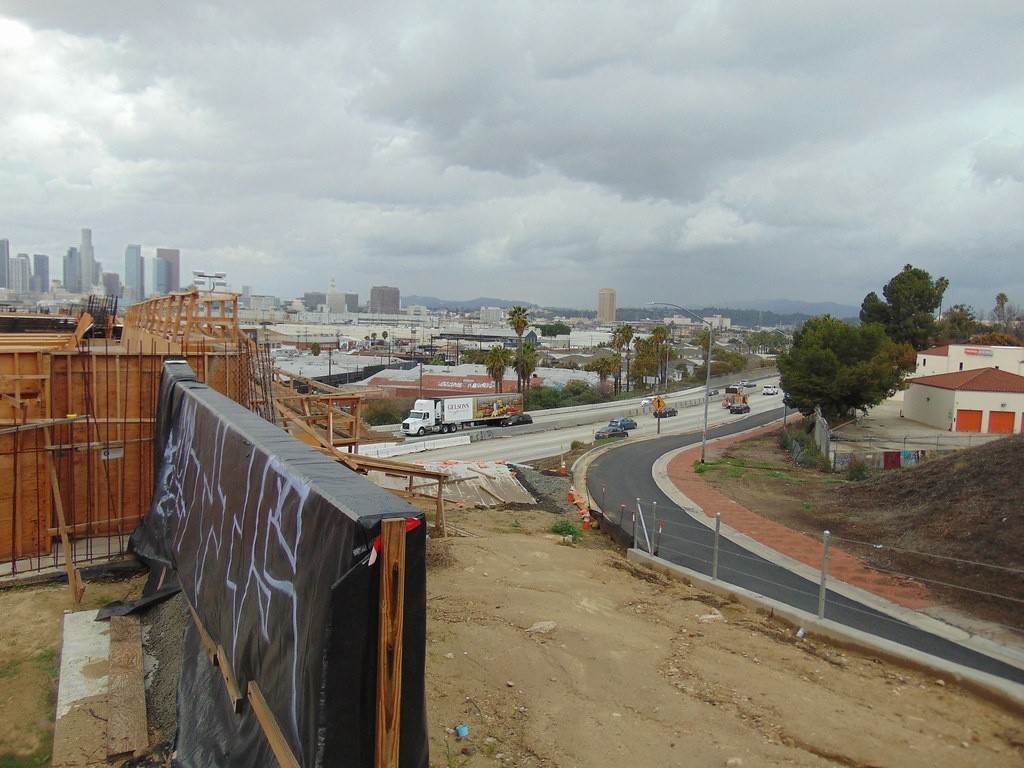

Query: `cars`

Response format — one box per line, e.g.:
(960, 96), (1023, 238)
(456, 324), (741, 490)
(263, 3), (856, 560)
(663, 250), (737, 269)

(729, 403), (750, 414)
(739, 382), (757, 388)
(739, 380), (750, 386)
(653, 407), (678, 418)
(595, 425), (628, 441)
(729, 384), (742, 391)
(499, 414), (533, 427)
(706, 389), (720, 396)
(608, 417), (637, 431)
(641, 396), (656, 407)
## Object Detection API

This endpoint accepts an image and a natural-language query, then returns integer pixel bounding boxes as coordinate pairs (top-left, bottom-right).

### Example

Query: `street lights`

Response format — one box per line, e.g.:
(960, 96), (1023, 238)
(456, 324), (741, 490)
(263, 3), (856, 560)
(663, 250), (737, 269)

(645, 317), (670, 394)
(646, 300), (713, 472)
(192, 268), (227, 329)
(774, 327), (793, 431)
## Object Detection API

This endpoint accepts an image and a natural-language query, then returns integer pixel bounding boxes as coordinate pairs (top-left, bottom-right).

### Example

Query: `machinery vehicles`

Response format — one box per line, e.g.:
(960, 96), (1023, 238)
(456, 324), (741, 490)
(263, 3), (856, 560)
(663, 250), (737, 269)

(722, 390), (749, 408)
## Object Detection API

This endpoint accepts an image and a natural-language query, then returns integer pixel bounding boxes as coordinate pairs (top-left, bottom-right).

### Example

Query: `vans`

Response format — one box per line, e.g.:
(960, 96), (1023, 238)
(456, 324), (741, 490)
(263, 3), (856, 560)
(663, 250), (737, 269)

(763, 384), (778, 395)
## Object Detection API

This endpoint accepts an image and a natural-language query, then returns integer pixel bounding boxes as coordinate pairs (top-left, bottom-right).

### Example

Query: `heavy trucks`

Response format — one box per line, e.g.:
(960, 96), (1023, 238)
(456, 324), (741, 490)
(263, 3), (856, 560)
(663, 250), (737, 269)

(397, 391), (524, 436)
